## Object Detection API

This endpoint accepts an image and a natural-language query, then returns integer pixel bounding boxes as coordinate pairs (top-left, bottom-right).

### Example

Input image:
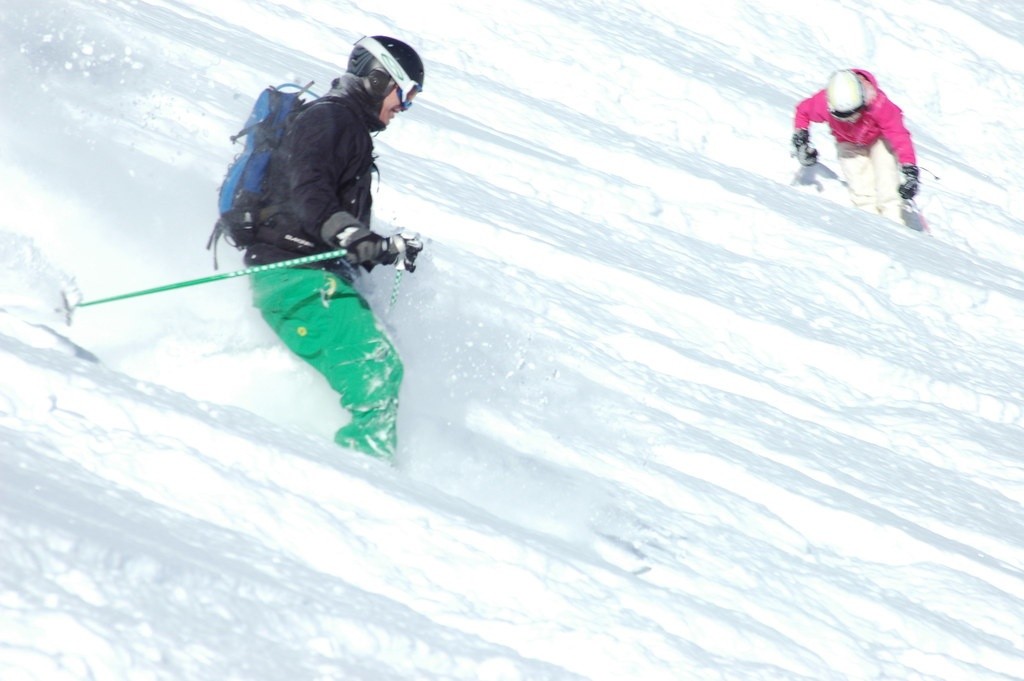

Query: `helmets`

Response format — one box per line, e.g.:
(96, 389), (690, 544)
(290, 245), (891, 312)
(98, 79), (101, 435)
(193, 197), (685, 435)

(827, 69), (864, 124)
(347, 36), (424, 90)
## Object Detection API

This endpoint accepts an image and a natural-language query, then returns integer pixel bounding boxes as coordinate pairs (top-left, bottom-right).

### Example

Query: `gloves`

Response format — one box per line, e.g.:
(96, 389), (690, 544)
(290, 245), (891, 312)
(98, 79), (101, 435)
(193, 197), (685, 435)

(793, 132), (817, 166)
(323, 210), (388, 264)
(376, 231), (423, 272)
(900, 166), (920, 200)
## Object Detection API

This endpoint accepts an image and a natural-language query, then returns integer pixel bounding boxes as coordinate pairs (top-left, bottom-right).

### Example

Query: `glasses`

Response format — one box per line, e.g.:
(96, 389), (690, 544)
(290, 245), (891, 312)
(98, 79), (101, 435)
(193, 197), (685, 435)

(829, 105), (864, 118)
(356, 35), (420, 111)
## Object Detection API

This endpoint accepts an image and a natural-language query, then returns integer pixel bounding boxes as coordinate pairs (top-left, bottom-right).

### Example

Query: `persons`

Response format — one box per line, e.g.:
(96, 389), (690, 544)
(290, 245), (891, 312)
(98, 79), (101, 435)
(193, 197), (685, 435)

(791, 69), (920, 230)
(248, 36), (425, 468)
